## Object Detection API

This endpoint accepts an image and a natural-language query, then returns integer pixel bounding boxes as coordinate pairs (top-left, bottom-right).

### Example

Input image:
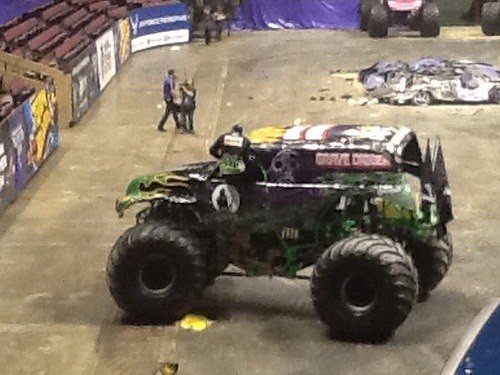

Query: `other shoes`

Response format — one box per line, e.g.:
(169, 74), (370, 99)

(158, 128), (167, 132)
(176, 125), (183, 129)
(184, 130), (196, 134)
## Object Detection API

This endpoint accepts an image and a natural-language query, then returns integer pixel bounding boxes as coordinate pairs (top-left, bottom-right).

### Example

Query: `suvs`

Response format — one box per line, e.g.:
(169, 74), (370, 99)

(360, 0), (441, 39)
(100, 127), (454, 348)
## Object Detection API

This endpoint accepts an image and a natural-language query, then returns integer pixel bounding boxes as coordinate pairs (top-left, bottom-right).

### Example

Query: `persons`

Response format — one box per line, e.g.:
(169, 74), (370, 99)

(156, 68), (183, 132)
(178, 78), (197, 135)
(208, 123), (257, 184)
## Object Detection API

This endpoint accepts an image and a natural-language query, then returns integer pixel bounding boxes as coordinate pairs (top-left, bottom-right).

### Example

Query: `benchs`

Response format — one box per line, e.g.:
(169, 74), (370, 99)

(0, 0), (183, 122)
